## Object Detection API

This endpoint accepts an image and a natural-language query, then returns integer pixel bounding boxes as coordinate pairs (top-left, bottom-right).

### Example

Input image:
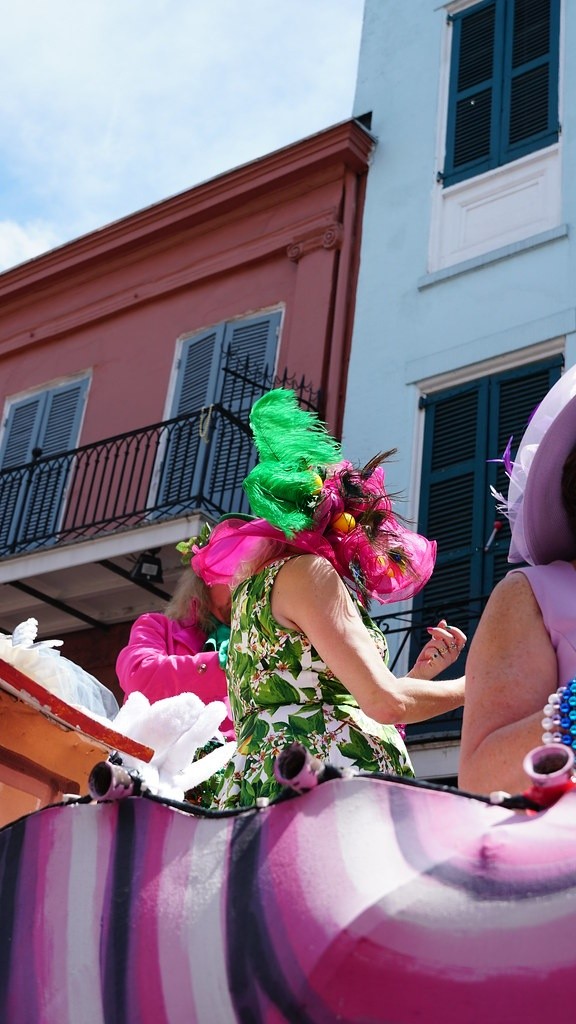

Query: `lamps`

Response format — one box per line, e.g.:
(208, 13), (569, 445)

(128, 546), (164, 584)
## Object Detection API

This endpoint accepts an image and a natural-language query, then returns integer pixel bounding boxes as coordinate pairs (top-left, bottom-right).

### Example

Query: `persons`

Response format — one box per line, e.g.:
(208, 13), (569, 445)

(455, 359), (576, 811)
(203, 459), (467, 816)
(116, 557), (238, 742)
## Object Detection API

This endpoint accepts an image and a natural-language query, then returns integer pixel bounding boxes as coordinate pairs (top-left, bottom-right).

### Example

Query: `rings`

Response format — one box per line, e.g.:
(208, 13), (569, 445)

(449, 642), (456, 650)
(432, 635), (443, 641)
(439, 646), (448, 655)
(432, 653), (439, 659)
(461, 644), (465, 650)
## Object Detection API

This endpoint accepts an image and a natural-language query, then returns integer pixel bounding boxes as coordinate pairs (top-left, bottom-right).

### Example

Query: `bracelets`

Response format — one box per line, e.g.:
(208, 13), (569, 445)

(541, 675), (576, 783)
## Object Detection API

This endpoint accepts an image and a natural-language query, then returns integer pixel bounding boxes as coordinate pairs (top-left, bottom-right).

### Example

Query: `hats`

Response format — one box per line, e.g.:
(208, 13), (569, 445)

(192, 387), (437, 604)
(487, 364), (576, 566)
(177, 513), (262, 565)
